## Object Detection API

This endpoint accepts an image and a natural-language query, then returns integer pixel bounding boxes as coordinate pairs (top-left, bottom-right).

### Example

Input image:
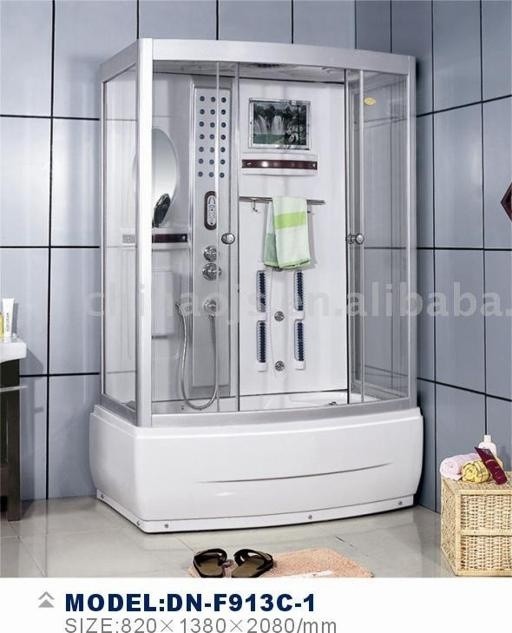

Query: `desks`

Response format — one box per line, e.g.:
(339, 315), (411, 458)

(1, 359), (27, 522)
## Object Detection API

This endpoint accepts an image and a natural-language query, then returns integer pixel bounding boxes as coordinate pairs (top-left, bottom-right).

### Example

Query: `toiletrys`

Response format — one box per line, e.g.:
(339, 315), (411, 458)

(478, 434), (497, 460)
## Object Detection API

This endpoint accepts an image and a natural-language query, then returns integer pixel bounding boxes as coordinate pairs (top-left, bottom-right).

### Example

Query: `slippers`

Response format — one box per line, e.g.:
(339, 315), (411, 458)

(193, 548), (227, 578)
(231, 549), (274, 578)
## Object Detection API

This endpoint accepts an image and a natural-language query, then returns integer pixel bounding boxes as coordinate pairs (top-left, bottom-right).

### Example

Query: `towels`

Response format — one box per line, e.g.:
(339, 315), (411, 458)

(264, 197), (313, 271)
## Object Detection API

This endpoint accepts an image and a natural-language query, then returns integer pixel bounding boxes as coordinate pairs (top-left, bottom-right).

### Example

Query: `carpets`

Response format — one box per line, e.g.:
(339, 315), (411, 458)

(188, 547), (374, 578)
